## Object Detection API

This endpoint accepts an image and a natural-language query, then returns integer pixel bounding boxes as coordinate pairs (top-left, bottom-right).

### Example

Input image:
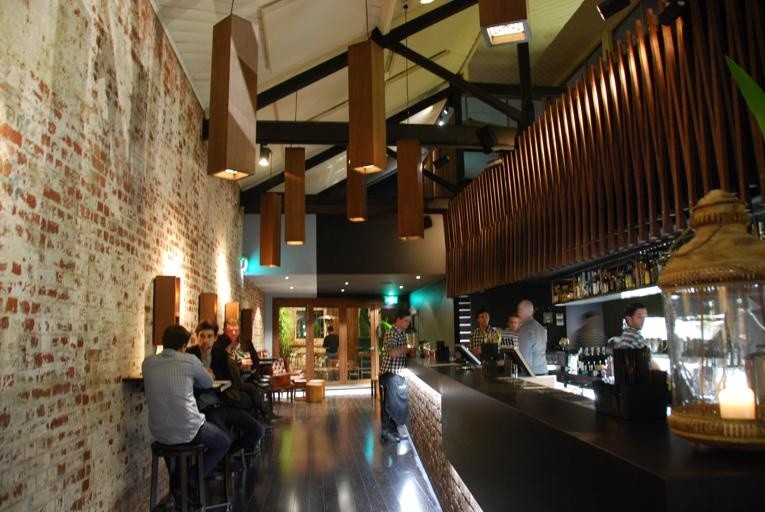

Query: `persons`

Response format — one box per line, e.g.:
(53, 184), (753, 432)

(618, 303), (660, 371)
(574, 311), (607, 352)
(323, 326), (339, 380)
(469, 308), (501, 361)
(501, 314), (519, 377)
(515, 299), (548, 375)
(380, 309), (416, 443)
(142, 325), (232, 510)
(186, 320), (265, 470)
(216, 318), (285, 420)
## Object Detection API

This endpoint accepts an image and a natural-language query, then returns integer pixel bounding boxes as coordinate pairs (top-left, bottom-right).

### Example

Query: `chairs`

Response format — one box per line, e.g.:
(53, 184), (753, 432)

(266, 358), (305, 404)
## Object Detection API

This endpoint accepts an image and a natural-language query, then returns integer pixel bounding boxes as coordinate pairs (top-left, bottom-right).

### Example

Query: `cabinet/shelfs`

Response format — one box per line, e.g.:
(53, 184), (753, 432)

(551, 214), (765, 379)
(153, 275), (179, 347)
(225, 301), (239, 324)
(197, 293), (217, 328)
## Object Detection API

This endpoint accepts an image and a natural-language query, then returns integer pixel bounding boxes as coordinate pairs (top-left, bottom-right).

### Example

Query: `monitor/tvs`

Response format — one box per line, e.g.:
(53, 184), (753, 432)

(500, 346), (536, 377)
(455, 344), (482, 366)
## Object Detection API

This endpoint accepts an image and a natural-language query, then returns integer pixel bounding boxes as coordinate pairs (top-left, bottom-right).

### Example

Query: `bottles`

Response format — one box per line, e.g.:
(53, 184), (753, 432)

(576, 346), (610, 377)
(552, 249), (665, 303)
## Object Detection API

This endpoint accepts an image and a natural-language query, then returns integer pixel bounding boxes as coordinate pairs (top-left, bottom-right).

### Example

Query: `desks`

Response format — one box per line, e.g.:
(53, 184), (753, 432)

(123, 377), (232, 397)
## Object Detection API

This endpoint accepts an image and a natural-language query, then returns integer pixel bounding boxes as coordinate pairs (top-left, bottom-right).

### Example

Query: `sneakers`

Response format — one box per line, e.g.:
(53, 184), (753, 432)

(254, 411), (262, 420)
(171, 482), (200, 509)
(223, 453), (233, 465)
(265, 412), (283, 419)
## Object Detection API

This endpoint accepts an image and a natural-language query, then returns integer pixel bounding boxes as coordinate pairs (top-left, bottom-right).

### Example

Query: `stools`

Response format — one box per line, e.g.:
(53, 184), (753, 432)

(262, 373), (271, 380)
(259, 378), (269, 383)
(307, 382), (323, 402)
(309, 378), (326, 399)
(150, 442), (209, 512)
(257, 383), (272, 416)
(232, 449), (260, 458)
(209, 445), (231, 511)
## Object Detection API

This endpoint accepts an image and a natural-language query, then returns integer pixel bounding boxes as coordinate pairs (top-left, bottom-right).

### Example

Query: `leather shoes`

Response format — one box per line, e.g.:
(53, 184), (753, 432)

(380, 430), (399, 442)
(390, 432), (407, 440)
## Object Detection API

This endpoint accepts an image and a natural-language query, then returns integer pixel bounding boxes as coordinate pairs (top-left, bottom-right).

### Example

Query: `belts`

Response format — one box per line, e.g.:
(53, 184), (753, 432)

(212, 403), (221, 408)
